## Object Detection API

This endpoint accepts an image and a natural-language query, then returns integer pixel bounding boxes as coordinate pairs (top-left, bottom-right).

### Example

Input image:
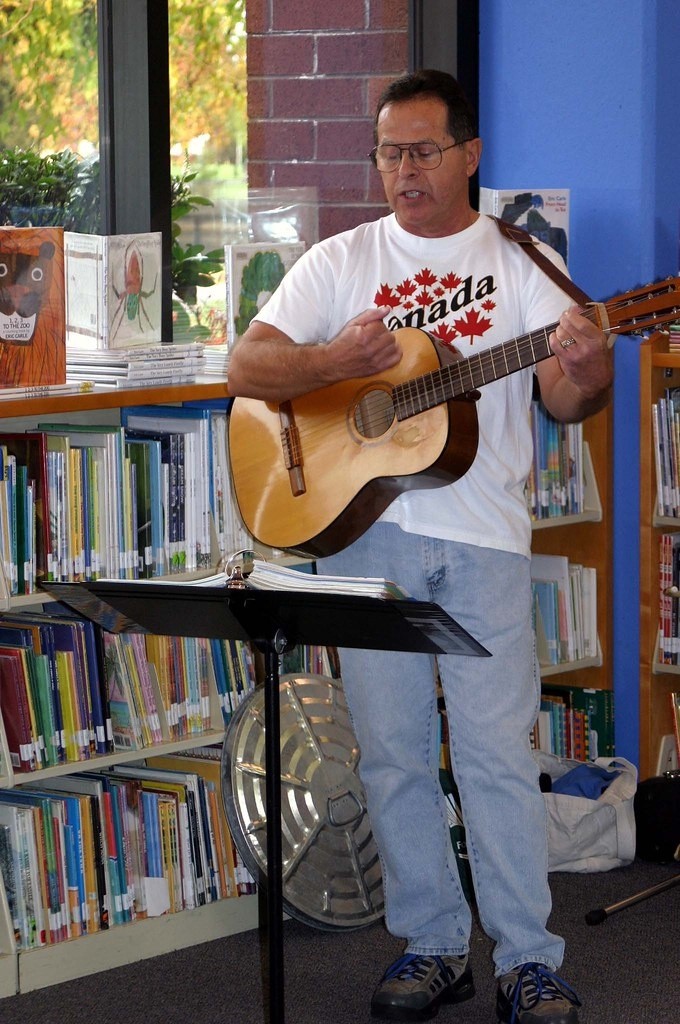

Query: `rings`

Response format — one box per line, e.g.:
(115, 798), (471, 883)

(561, 338), (575, 348)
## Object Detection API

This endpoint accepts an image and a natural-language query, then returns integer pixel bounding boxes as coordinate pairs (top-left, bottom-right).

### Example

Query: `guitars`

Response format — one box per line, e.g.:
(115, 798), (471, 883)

(228, 269), (680, 559)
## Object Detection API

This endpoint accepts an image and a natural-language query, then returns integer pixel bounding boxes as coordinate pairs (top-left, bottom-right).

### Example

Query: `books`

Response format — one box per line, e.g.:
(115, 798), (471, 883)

(0, 227), (340, 959)
(479, 187), (569, 267)
(529, 552), (598, 666)
(525, 399), (584, 522)
(434, 682), (615, 775)
(650, 324), (680, 752)
(98, 551), (414, 602)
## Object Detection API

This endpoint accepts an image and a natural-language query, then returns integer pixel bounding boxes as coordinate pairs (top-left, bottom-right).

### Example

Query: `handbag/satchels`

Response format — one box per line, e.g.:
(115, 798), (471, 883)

(530, 749), (638, 874)
(633, 770), (680, 862)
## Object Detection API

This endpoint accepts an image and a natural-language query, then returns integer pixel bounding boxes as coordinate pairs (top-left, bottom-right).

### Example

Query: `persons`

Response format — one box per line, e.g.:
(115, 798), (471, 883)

(226, 71), (613, 1024)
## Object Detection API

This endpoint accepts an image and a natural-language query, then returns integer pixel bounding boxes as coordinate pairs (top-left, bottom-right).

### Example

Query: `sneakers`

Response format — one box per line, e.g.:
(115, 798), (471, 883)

(496, 963), (583, 1024)
(371, 953), (476, 1022)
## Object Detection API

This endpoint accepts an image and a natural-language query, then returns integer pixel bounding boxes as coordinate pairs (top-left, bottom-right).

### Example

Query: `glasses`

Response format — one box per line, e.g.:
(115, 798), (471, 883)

(367, 139), (472, 170)
